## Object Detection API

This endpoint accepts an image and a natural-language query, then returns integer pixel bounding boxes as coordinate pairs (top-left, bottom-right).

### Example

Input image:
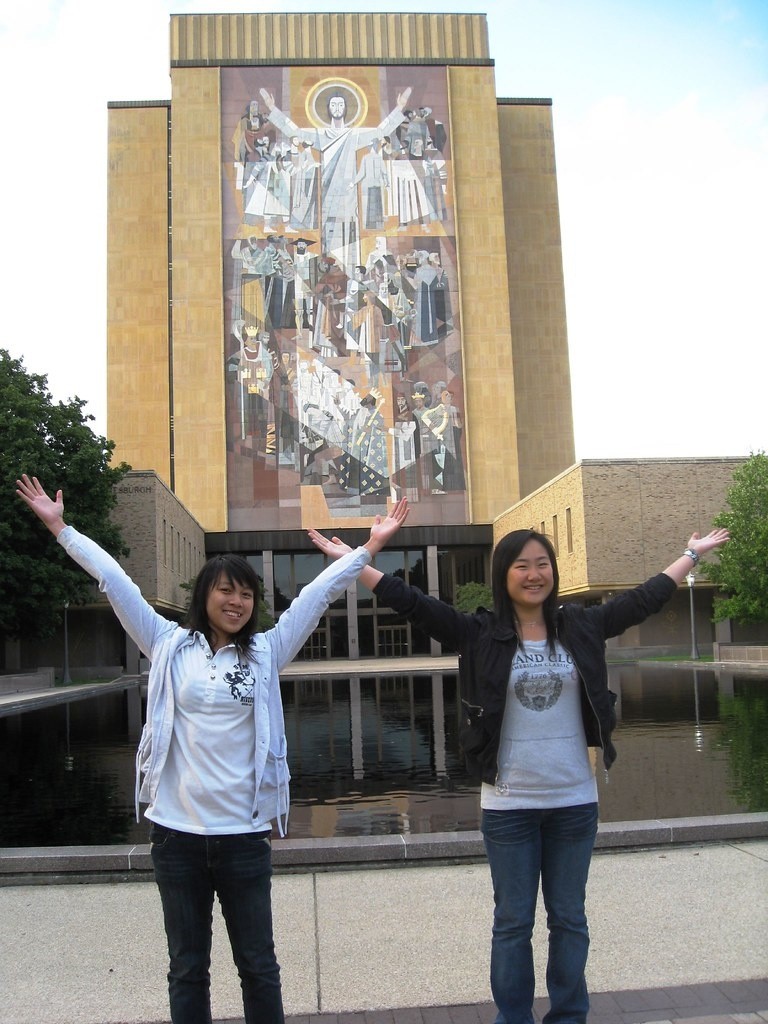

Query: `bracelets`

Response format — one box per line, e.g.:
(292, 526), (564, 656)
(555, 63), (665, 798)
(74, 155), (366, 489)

(683, 548), (698, 567)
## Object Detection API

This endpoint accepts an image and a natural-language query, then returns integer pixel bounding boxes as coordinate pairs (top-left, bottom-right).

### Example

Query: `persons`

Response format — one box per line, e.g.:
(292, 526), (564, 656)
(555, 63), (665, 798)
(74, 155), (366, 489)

(308, 526), (731, 1024)
(16, 474), (411, 1024)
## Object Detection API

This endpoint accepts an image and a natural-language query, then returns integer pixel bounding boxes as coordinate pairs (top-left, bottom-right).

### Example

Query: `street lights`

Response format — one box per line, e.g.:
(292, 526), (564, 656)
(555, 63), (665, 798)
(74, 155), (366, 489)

(693, 669), (707, 753)
(62, 598), (73, 685)
(685, 570), (702, 660)
(64, 702), (77, 772)
